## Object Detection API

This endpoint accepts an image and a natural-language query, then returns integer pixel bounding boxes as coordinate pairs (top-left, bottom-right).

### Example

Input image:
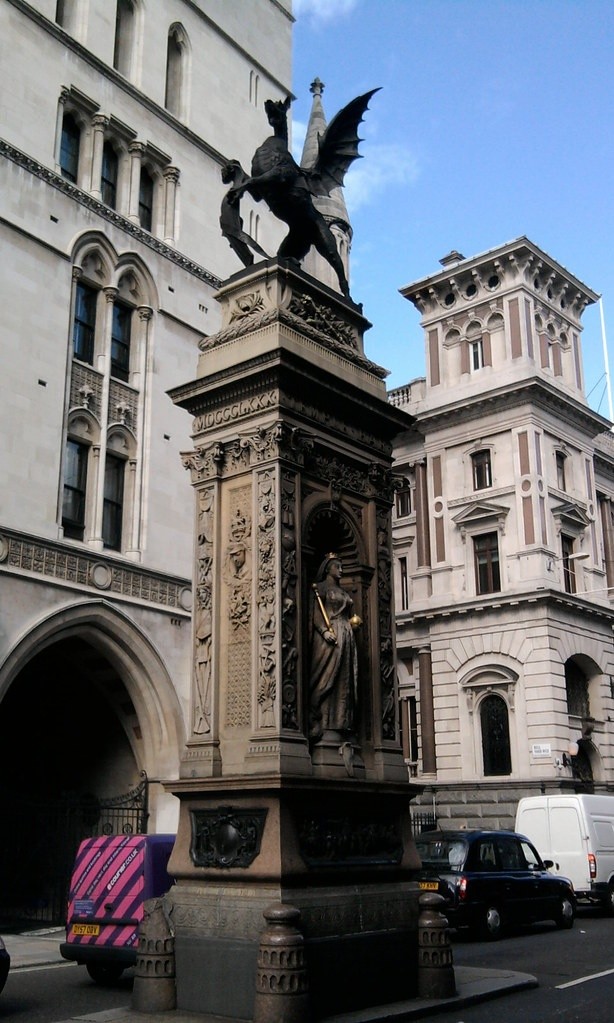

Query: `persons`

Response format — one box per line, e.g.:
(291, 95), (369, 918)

(230, 547), (245, 568)
(310, 556), (357, 735)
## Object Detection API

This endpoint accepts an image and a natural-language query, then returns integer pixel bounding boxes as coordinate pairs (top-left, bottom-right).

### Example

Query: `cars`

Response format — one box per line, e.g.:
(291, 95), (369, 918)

(415, 830), (572, 944)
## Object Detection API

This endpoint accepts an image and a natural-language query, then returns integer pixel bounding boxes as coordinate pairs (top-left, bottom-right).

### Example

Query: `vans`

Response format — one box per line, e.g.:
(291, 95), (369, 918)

(514, 793), (614, 916)
(59, 833), (178, 985)
